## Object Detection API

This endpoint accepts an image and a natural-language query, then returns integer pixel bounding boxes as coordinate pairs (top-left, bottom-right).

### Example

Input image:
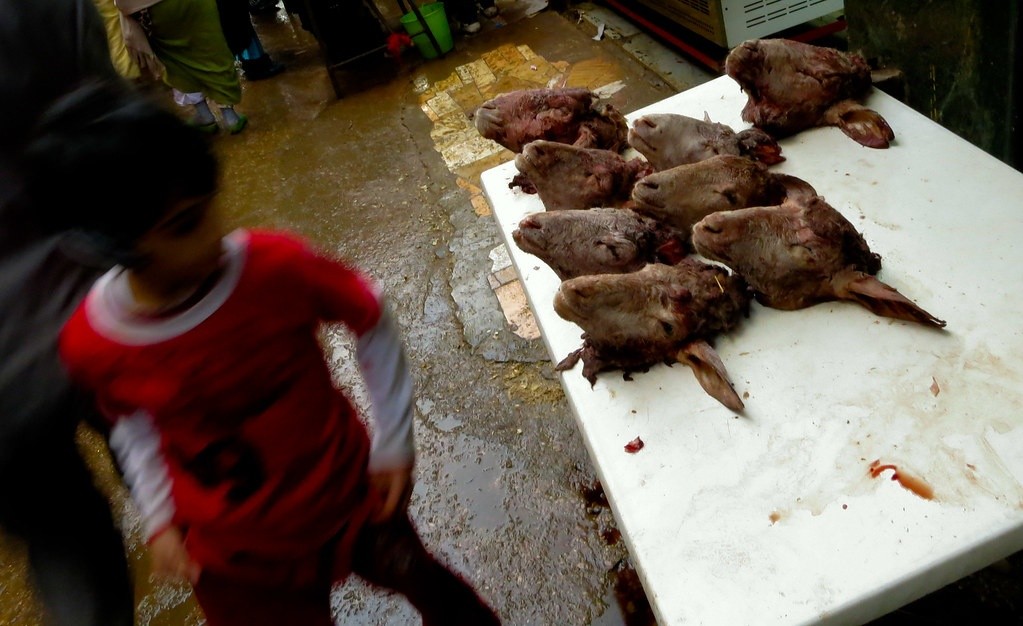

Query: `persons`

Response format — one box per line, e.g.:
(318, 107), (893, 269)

(0, 0), (159, 626)
(84, 0), (401, 131)
(44, 80), (503, 626)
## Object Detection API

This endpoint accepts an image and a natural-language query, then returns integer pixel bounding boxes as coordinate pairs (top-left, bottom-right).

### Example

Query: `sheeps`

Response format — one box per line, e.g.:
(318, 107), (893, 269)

(551, 264), (747, 411)
(691, 192), (948, 329)
(723, 37), (896, 148)
(473, 85), (629, 155)
(631, 153), (815, 231)
(507, 140), (656, 212)
(626, 108), (786, 169)
(512, 204), (691, 282)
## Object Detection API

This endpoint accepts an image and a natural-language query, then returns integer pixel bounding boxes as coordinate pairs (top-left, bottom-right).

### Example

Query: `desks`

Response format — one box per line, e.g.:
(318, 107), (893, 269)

(480, 76), (1023, 626)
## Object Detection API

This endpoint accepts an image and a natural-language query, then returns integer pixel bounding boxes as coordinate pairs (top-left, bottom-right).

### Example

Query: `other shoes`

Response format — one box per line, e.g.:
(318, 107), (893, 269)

(453, 21), (481, 35)
(479, 5), (498, 17)
(243, 55), (273, 80)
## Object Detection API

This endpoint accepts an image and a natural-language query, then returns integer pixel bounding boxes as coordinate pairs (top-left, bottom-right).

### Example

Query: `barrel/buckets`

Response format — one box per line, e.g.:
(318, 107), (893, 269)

(400, 2), (454, 60)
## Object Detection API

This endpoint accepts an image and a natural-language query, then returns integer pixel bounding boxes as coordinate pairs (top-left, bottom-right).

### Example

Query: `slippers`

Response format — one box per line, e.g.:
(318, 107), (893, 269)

(186, 117), (218, 133)
(228, 114), (246, 131)
